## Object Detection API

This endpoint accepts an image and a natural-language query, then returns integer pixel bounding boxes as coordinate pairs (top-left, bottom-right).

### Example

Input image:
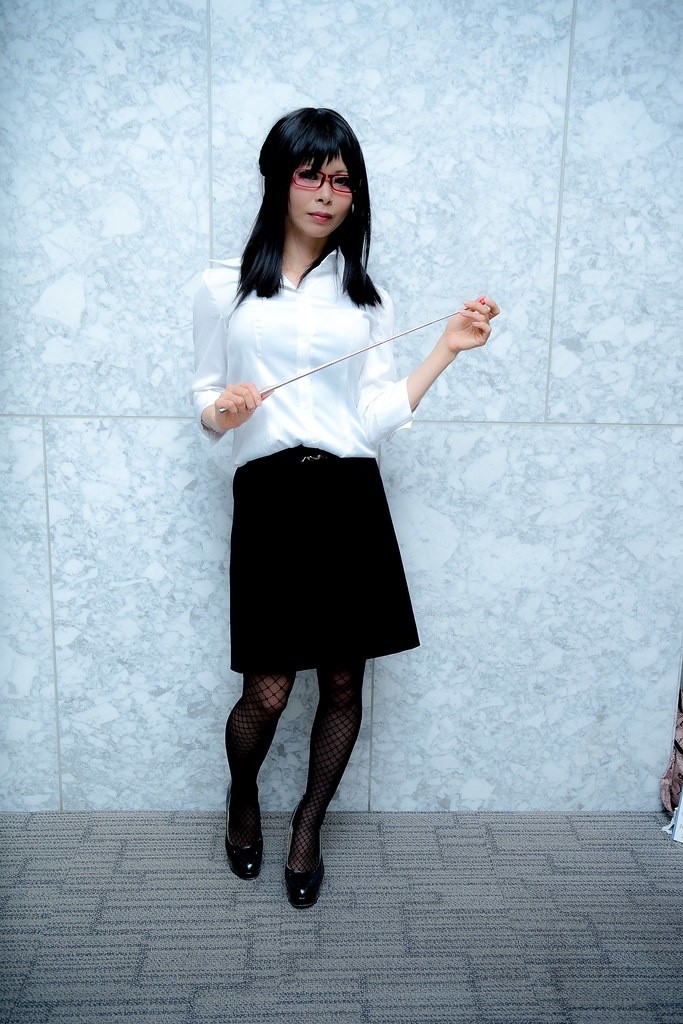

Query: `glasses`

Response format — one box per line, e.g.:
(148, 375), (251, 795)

(290, 168), (354, 193)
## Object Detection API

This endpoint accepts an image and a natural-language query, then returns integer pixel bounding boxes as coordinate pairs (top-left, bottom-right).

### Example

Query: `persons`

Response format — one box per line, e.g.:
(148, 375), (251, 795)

(191, 109), (499, 908)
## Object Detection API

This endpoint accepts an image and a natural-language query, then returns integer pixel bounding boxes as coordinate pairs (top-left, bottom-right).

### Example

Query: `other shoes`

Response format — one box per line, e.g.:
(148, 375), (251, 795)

(285, 810), (325, 910)
(225, 780), (263, 880)
